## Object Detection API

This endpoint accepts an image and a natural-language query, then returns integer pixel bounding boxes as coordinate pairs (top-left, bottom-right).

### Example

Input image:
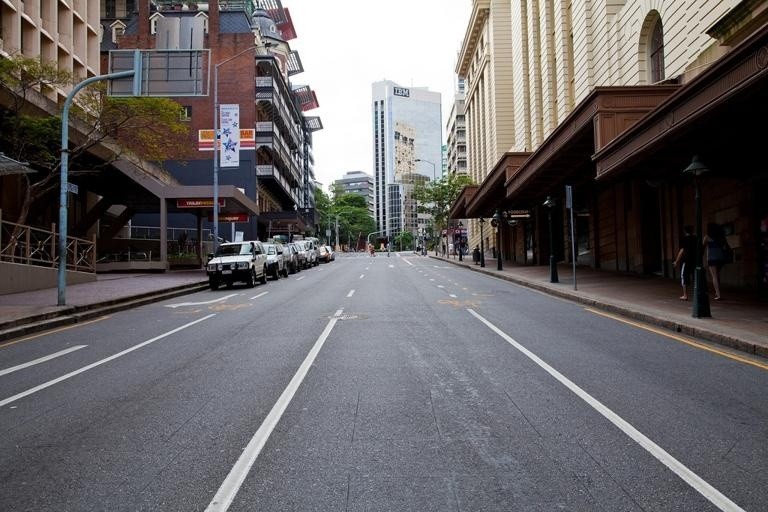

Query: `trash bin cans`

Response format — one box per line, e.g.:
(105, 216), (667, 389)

(473, 248), (480, 261)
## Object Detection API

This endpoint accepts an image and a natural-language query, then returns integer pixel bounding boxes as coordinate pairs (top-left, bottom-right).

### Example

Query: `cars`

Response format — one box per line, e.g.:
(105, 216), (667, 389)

(207, 235), (336, 293)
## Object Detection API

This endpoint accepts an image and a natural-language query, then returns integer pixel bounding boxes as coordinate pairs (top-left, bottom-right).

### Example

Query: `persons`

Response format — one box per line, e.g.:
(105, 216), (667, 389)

(368, 241), (376, 258)
(672, 225), (700, 304)
(702, 224), (730, 301)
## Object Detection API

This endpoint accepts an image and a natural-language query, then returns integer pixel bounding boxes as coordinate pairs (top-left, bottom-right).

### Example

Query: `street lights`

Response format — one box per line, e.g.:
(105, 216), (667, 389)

(335, 211), (353, 251)
(479, 215), (487, 268)
(492, 209), (504, 270)
(368, 226), (396, 245)
(543, 193), (561, 282)
(421, 228), (427, 256)
(414, 158), (438, 257)
(55, 47), (145, 309)
(681, 153), (717, 319)
(209, 40), (280, 245)
(458, 221), (463, 262)
